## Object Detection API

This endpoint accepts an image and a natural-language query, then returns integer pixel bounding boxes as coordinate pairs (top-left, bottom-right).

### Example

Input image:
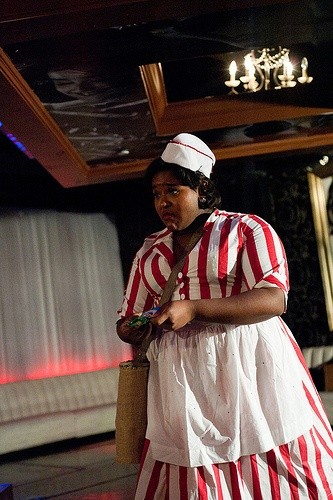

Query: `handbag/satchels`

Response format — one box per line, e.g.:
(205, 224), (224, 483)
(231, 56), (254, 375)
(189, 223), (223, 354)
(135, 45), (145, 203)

(114, 360), (151, 465)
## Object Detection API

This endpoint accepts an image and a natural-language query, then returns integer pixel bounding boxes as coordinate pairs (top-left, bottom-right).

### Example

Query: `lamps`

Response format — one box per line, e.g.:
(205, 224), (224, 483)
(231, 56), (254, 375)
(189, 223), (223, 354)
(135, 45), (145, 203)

(225, 47), (314, 94)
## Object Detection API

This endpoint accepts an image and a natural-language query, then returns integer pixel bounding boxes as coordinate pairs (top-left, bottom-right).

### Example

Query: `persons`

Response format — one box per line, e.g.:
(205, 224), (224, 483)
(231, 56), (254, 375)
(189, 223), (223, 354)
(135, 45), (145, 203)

(115, 132), (333, 500)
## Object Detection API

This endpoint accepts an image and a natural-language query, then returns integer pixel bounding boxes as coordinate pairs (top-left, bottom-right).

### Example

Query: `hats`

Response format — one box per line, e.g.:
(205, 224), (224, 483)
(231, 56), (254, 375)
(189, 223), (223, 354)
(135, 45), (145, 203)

(160, 132), (216, 179)
